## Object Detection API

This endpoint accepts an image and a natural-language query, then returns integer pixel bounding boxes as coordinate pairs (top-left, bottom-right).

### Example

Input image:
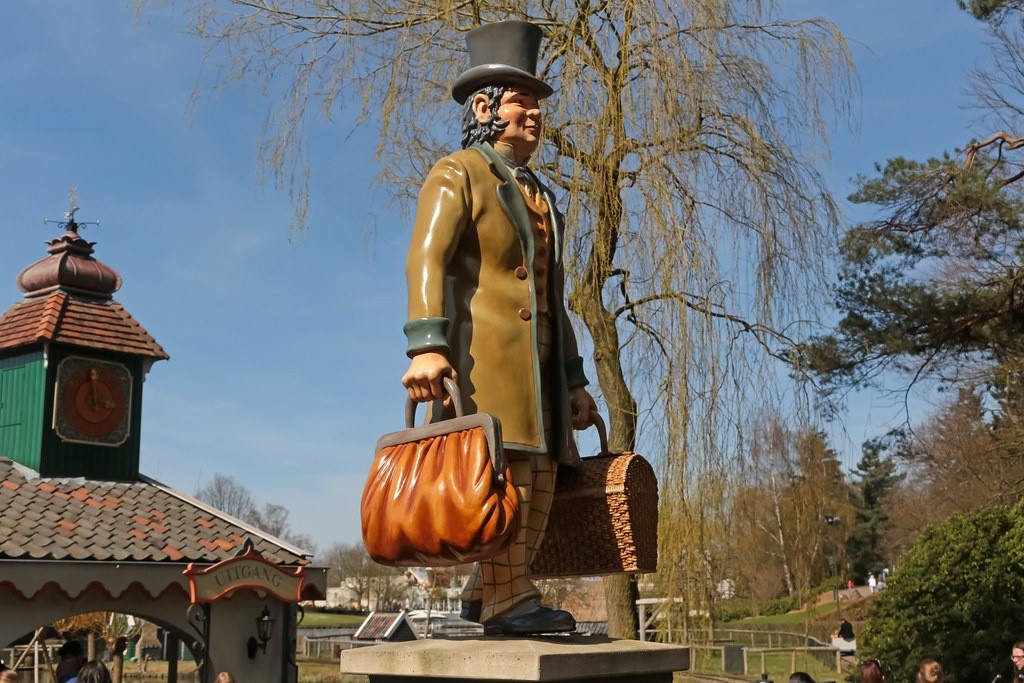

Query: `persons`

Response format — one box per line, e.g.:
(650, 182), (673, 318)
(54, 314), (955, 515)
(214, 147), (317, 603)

(789, 640), (1024, 683)
(0, 639), (236, 683)
(868, 574), (877, 593)
(847, 580), (855, 594)
(838, 618), (854, 640)
(402, 20), (597, 636)
